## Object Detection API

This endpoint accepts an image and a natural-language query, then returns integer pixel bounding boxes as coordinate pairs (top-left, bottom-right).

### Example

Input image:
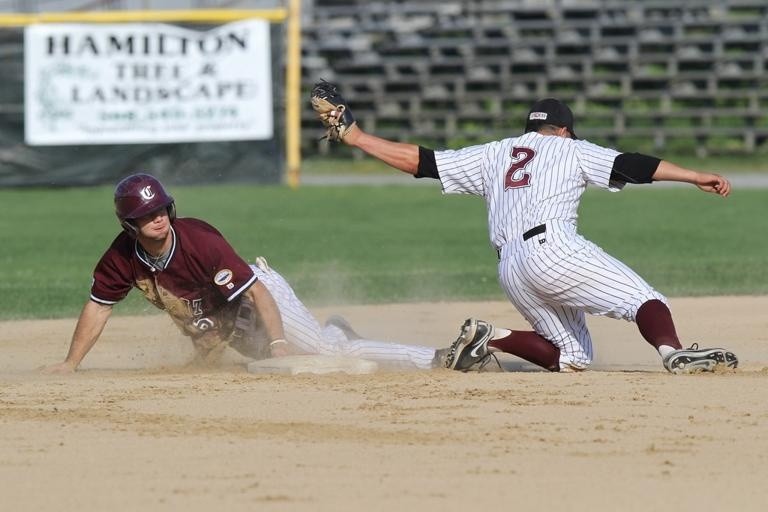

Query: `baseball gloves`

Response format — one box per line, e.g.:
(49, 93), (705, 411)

(311, 79), (356, 144)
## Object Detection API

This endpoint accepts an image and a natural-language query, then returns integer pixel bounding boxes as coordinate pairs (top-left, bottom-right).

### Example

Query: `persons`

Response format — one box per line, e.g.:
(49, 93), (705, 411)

(310, 82), (738, 373)
(61, 174), (452, 375)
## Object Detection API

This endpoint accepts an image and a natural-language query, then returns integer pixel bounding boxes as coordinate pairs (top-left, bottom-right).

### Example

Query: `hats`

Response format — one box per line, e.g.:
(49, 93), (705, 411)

(525, 98), (577, 139)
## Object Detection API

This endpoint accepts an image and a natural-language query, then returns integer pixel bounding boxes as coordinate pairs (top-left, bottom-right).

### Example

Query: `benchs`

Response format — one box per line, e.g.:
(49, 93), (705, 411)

(279, 1), (768, 162)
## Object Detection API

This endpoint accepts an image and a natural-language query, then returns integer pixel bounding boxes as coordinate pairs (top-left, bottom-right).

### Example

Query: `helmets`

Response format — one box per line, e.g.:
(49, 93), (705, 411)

(113, 173), (177, 239)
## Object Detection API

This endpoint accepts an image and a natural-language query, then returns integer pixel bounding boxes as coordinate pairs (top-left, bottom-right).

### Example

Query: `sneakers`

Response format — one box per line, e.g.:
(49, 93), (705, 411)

(324, 313), (365, 341)
(445, 316), (494, 374)
(663, 347), (738, 379)
